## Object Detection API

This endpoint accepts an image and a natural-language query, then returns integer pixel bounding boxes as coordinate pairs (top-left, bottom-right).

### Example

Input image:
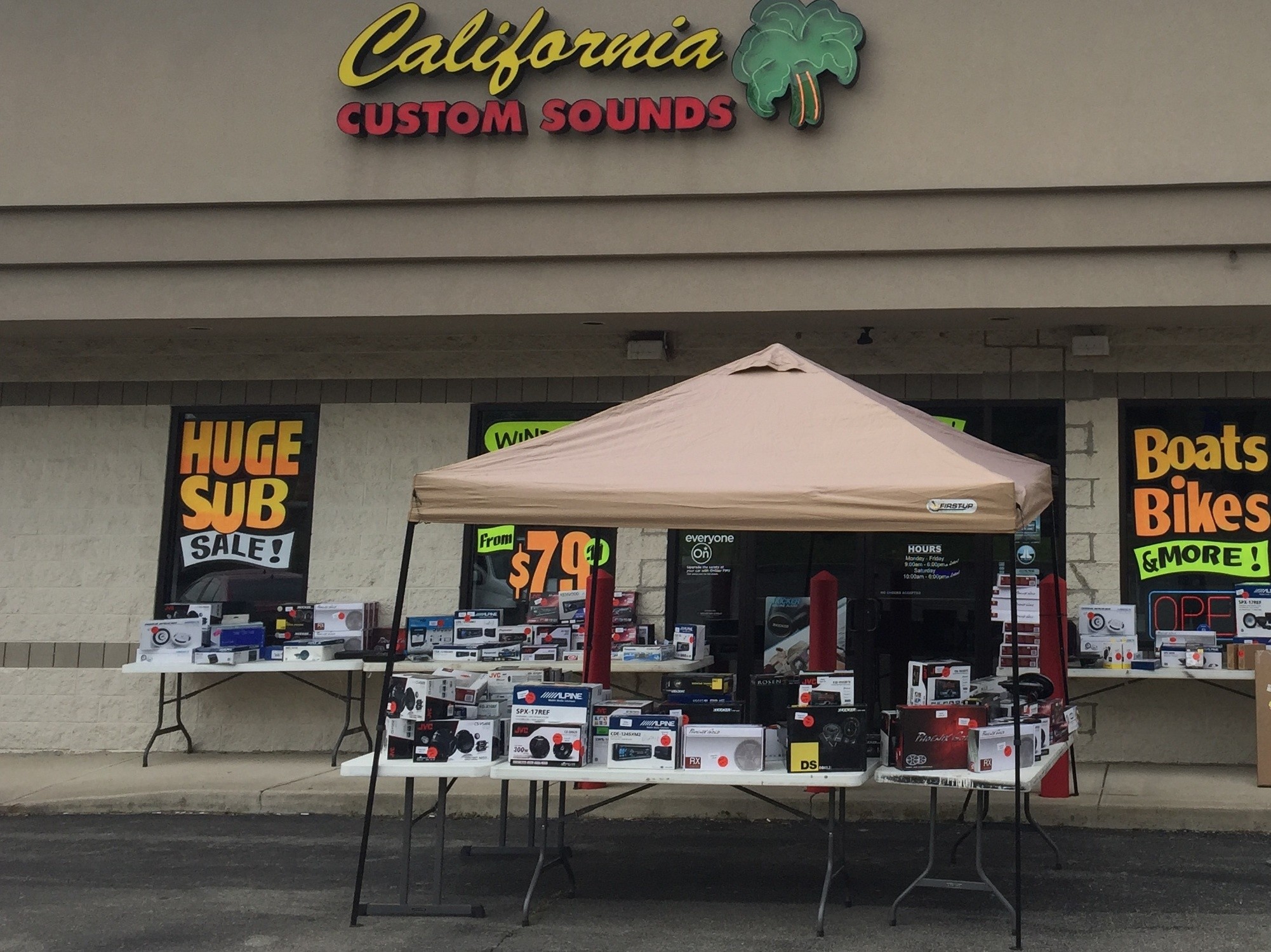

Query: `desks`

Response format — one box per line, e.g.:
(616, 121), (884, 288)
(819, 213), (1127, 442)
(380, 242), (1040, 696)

(340, 748), (572, 918)
(875, 735), (1075, 935)
(491, 744), (883, 936)
(123, 659), (372, 768)
(135, 570), (1271, 786)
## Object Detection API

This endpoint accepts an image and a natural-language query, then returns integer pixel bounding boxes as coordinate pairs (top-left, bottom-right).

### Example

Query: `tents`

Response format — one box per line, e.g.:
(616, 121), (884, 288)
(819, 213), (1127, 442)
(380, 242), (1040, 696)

(351, 343), (1079, 952)
